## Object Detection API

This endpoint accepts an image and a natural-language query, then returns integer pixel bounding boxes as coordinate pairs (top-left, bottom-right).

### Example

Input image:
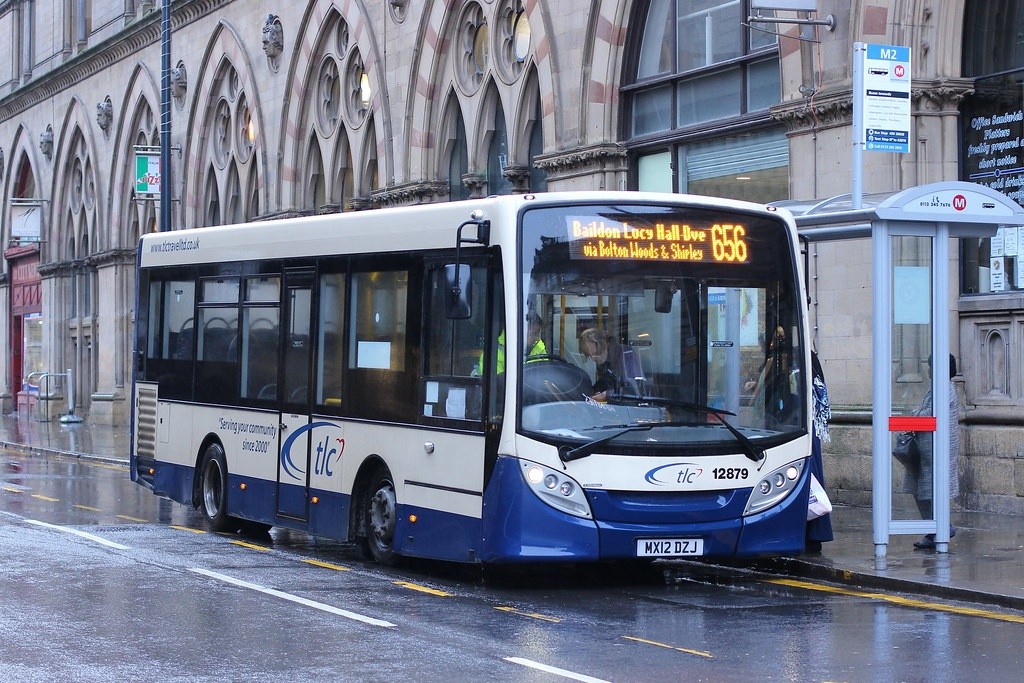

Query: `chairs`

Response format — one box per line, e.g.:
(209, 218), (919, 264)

(225, 319), (238, 339)
(204, 317), (233, 362)
(324, 321), (340, 360)
(258, 384), (287, 401)
(177, 318), (207, 359)
(231, 335), (261, 363)
(291, 385), (308, 403)
(250, 319), (276, 342)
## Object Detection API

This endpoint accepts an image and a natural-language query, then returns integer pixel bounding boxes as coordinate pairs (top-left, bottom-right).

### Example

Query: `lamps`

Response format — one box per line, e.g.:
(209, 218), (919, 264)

(797, 85), (814, 98)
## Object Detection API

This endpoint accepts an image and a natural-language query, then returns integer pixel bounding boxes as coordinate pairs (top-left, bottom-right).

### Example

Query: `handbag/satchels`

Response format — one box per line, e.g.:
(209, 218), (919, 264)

(893, 432), (921, 473)
(806, 471), (832, 521)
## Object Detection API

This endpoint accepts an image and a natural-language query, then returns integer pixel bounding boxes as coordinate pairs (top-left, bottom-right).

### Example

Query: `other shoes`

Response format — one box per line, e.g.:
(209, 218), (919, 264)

(805, 540), (822, 552)
(913, 537), (936, 548)
(950, 528), (955, 538)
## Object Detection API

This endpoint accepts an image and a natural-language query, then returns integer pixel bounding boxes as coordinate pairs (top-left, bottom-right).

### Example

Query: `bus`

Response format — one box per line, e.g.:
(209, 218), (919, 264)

(129, 190), (813, 565)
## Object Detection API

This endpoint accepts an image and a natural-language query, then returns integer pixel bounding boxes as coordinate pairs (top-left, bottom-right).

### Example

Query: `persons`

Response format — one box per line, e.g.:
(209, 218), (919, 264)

(902, 352), (959, 549)
(744, 326), (834, 554)
(479, 312), (548, 376)
(579, 328), (647, 402)
